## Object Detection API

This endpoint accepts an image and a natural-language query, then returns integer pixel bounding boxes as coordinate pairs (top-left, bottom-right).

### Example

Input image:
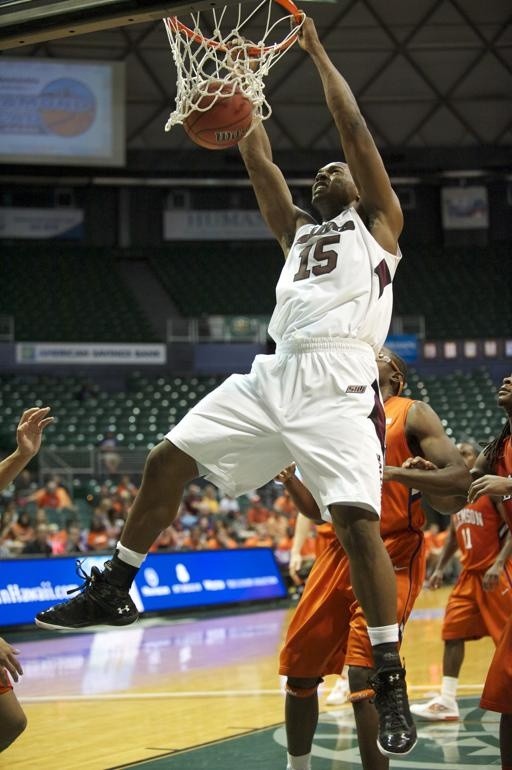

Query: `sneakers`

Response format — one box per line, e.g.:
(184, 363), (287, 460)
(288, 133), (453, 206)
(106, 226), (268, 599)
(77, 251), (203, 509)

(409, 696), (460, 720)
(374, 664), (418, 758)
(34, 566), (138, 632)
(325, 677), (351, 705)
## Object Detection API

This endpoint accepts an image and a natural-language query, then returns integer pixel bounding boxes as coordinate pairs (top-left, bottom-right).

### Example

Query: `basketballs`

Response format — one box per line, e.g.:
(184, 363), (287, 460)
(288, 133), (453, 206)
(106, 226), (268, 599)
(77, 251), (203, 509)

(183, 79), (252, 150)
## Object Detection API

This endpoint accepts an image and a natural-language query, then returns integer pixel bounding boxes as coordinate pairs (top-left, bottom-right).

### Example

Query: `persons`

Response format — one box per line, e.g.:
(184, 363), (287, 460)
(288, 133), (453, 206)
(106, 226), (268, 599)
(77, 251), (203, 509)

(36, 12), (418, 757)
(0, 466), (306, 554)
(277, 344), (512, 770)
(0, 405), (55, 752)
